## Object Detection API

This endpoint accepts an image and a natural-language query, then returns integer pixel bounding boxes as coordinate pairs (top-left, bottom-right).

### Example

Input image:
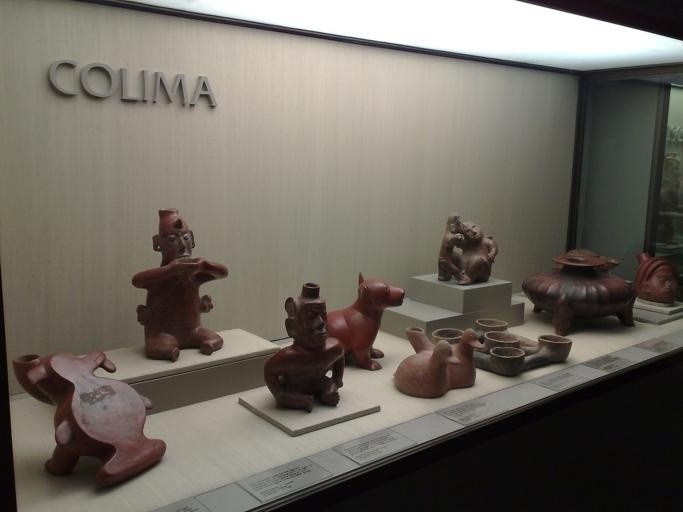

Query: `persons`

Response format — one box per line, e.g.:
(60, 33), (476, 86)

(262, 282), (345, 411)
(435, 213), (471, 285)
(634, 251), (679, 303)
(449, 222), (497, 282)
(130, 206), (230, 363)
